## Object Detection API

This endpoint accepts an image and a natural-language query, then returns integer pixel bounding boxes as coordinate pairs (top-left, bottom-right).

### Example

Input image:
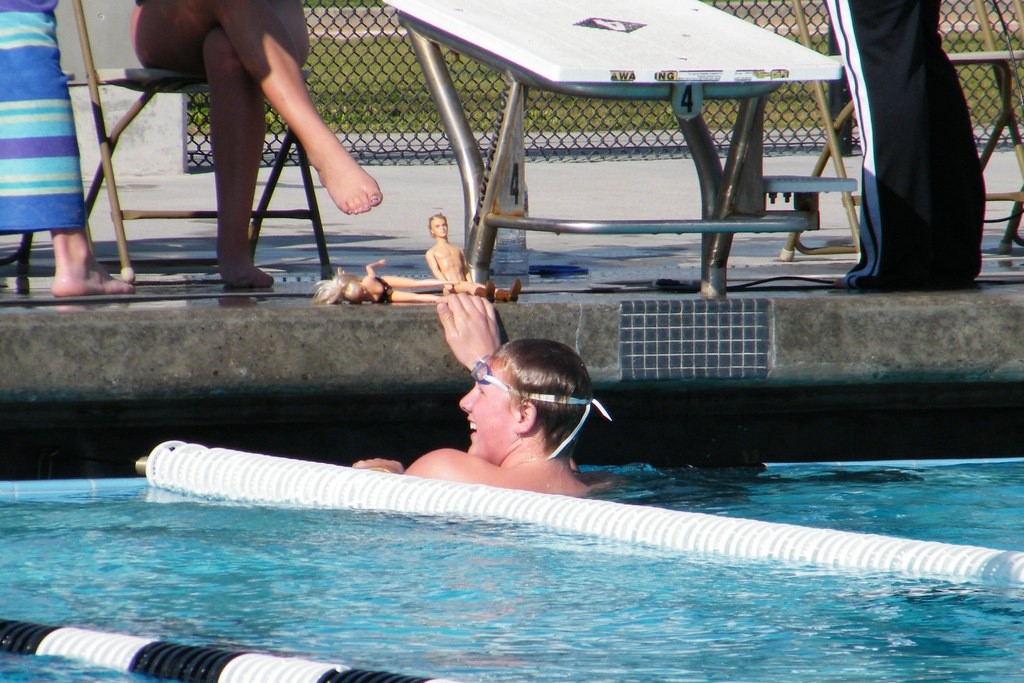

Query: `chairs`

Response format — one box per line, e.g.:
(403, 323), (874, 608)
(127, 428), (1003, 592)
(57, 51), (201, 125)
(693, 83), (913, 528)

(779, 1), (1024, 264)
(68, 1), (336, 286)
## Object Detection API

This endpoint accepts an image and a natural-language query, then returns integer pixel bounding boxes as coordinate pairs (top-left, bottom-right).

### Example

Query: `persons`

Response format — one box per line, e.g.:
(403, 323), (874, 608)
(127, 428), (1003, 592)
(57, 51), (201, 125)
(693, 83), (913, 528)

(318, 259), (460, 303)
(130, 1), (382, 290)
(829, 0), (983, 291)
(425, 214), (522, 302)
(0, 0), (135, 297)
(353, 293), (593, 496)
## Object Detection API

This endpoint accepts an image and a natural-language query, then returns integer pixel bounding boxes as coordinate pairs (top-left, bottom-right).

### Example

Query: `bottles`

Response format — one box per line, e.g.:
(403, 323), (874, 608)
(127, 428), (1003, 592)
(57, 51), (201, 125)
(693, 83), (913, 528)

(493, 181), (530, 275)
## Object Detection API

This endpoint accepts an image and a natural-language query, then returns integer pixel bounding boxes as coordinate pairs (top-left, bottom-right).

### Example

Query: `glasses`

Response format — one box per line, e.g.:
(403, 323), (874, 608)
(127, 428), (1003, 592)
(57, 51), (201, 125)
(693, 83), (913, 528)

(471, 354), (494, 386)
